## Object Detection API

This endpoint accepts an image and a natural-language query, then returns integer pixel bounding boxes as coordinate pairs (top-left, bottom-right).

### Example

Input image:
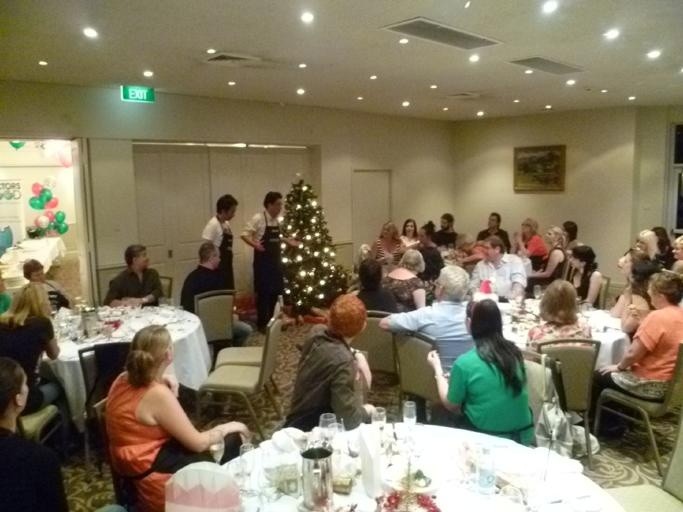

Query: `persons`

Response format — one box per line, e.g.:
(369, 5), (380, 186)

(102, 243), (163, 306)
(103, 324), (253, 511)
(0, 357), (71, 512)
(240, 190), (292, 331)
(0, 281), (74, 438)
(21, 260), (73, 311)
(0, 272), (12, 315)
(290, 293), (375, 431)
(424, 299), (532, 447)
(201, 192), (238, 295)
(180, 243), (250, 348)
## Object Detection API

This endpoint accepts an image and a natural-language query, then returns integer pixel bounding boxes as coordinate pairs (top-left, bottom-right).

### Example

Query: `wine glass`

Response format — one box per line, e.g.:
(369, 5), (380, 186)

(498, 282), (608, 337)
(206, 399), (446, 512)
(48, 296), (184, 346)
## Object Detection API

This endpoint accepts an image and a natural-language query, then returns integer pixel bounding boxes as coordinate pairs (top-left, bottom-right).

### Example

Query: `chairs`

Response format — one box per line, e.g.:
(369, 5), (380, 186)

(194, 289), (285, 442)
(350, 310), (456, 426)
(77, 341), (171, 506)
(516, 338), (681, 478)
(157, 275), (176, 304)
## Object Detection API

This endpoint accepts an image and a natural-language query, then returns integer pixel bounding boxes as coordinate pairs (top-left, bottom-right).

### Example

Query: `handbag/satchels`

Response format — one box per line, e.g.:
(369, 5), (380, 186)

(533, 353), (575, 463)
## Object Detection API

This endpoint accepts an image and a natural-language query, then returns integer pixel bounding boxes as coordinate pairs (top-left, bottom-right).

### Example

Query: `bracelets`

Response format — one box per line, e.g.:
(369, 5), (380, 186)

(142, 294), (150, 303)
(432, 371), (445, 381)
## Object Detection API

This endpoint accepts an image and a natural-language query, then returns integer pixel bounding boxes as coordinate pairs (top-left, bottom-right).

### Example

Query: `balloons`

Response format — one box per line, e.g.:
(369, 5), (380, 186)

(26, 173), (68, 237)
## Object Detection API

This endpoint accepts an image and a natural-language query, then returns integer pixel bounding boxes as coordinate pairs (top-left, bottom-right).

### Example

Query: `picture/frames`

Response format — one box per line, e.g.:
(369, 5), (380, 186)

(513, 143), (567, 192)
